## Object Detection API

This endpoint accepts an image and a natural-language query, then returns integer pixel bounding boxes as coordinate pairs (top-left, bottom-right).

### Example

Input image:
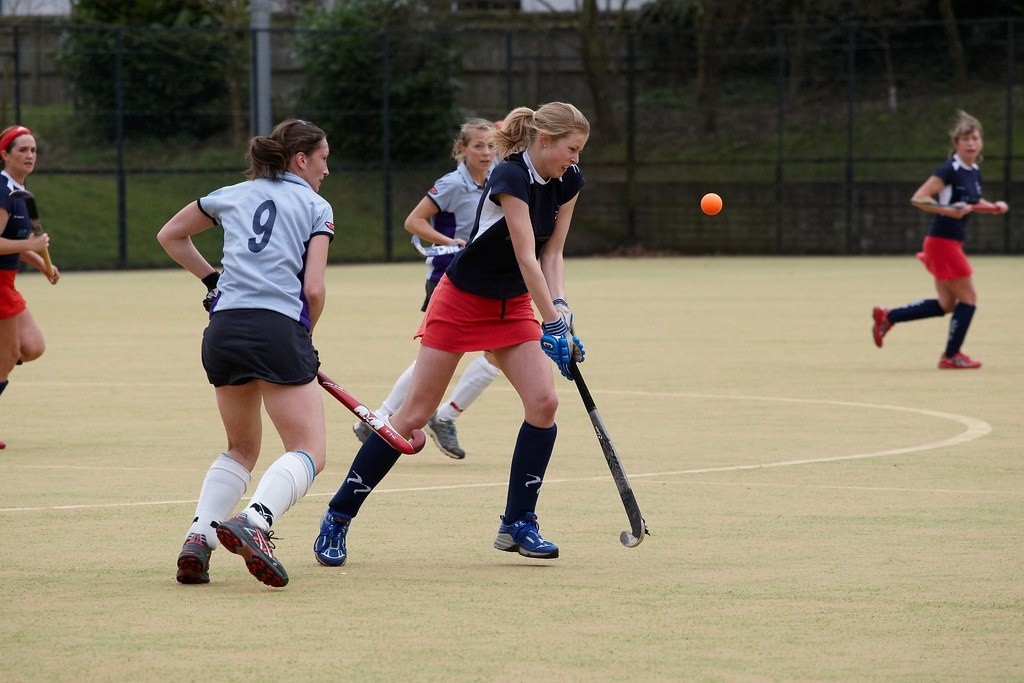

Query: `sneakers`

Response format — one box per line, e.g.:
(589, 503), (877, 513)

(493, 512), (560, 558)
(424, 410), (466, 459)
(353, 420), (373, 444)
(937, 351), (982, 369)
(872, 306), (893, 348)
(210, 512), (289, 587)
(313, 507), (351, 567)
(176, 532), (210, 584)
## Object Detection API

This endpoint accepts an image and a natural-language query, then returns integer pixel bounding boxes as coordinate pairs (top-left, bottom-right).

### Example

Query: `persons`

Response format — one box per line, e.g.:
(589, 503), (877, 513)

(871, 109), (1009, 369)
(351, 118), (504, 460)
(314, 102), (590, 566)
(0, 123), (61, 450)
(156, 120), (334, 586)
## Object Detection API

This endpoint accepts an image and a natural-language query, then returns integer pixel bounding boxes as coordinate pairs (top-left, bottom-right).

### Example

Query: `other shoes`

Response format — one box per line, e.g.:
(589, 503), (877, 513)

(0, 441), (6, 449)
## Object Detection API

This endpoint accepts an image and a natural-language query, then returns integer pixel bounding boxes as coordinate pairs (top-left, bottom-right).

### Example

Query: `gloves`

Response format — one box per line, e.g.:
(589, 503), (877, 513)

(539, 316), (585, 381)
(553, 298), (574, 334)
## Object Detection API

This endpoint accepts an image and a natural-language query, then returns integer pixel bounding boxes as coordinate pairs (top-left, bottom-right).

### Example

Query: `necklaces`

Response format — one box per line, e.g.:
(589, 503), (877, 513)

(474, 181), (484, 189)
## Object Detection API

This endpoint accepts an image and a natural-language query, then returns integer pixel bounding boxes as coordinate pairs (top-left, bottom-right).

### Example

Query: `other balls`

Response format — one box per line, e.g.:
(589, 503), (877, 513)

(701, 194), (725, 215)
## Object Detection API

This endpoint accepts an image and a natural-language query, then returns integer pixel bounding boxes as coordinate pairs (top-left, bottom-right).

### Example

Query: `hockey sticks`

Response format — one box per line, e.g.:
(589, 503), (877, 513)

(909, 198), (1003, 212)
(8, 190), (56, 277)
(565, 341), (644, 548)
(412, 236), (464, 257)
(312, 368), (427, 456)
